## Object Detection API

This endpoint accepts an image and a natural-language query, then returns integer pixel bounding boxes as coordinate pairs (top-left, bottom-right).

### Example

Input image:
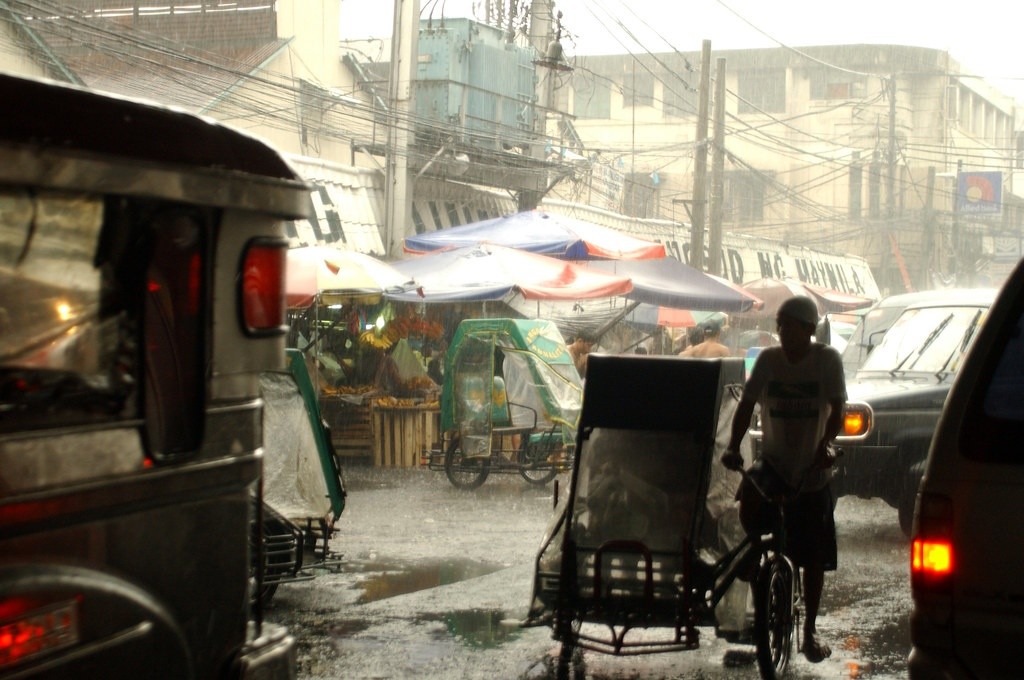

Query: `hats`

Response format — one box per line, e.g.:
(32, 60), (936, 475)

(702, 320), (721, 337)
(778, 296), (819, 327)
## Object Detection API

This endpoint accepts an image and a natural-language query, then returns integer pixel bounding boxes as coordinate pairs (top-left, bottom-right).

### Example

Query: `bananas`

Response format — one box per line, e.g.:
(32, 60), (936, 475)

(358, 315), (445, 350)
(321, 383), (413, 407)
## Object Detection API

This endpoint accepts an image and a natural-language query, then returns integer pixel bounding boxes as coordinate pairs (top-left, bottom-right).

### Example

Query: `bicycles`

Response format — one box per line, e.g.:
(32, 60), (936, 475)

(738, 462), (821, 680)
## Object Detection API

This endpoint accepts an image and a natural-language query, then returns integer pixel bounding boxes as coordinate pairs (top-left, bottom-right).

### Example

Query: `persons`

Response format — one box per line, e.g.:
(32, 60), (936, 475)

(718, 296), (849, 660)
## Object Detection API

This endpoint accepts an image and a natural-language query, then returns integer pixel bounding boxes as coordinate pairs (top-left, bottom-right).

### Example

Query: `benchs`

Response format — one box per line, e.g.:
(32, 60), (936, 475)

(464, 375), (538, 435)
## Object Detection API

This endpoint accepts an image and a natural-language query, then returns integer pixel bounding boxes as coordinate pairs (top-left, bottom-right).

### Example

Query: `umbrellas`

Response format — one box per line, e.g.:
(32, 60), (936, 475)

(279, 211), (876, 391)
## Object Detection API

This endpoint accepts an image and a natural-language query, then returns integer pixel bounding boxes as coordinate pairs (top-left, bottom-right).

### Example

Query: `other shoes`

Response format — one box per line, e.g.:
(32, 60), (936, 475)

(737, 548), (763, 581)
(801, 638), (824, 662)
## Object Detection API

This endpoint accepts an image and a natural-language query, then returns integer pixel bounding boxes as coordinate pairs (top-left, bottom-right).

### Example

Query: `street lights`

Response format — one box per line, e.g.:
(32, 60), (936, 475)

(359, 136), (471, 314)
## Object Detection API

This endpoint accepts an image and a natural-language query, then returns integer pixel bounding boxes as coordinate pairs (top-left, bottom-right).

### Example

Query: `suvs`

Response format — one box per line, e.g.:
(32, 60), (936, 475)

(0, 71), (304, 680)
(816, 257), (1023, 680)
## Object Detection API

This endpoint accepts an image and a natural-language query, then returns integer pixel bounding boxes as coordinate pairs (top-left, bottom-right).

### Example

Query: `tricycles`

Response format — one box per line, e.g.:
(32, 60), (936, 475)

(258, 347), (347, 585)
(420, 319), (583, 490)
